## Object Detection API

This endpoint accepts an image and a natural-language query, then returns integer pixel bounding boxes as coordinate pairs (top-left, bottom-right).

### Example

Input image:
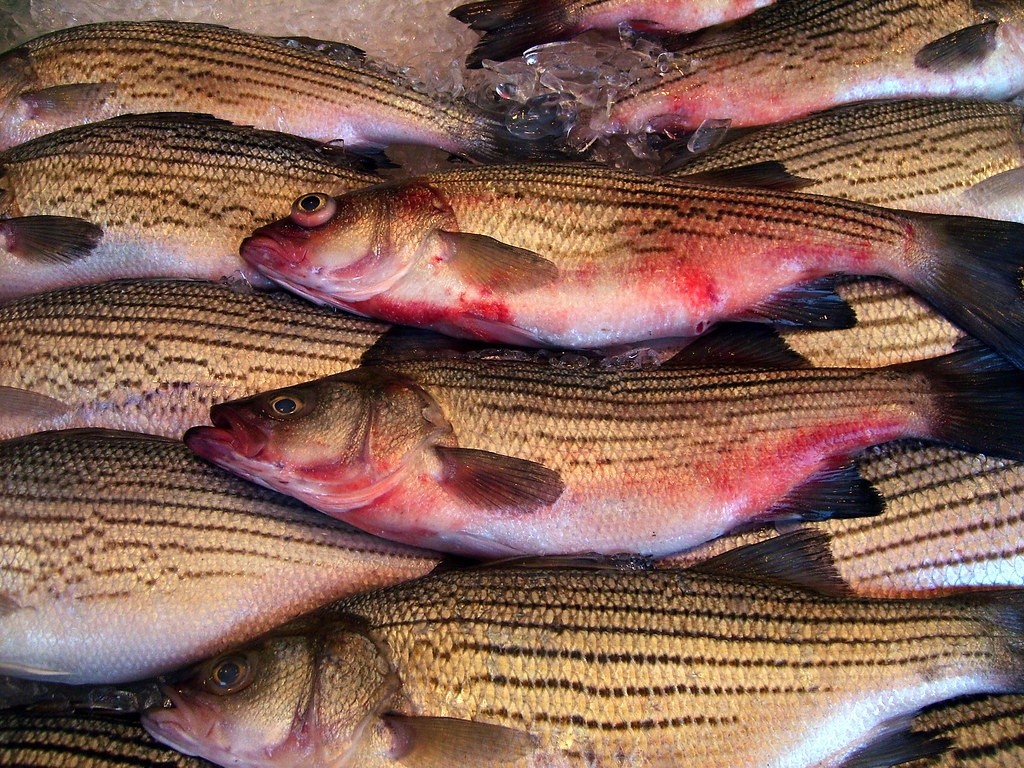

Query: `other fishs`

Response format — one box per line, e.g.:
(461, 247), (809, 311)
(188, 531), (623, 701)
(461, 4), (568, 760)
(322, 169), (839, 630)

(0, 0), (1024, 768)
